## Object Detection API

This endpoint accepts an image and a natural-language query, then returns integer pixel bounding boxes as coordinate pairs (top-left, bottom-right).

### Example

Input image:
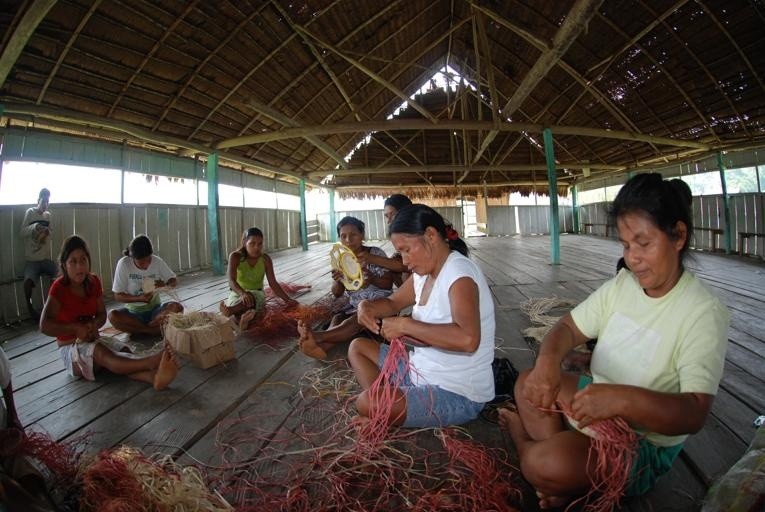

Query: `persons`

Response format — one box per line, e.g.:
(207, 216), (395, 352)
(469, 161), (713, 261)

(356, 194), (412, 288)
(496, 173), (730, 510)
(19, 234), (183, 392)
(348, 204), (496, 429)
(219, 227), (299, 331)
(19, 188), (60, 324)
(298, 216), (397, 362)
(0, 345), (57, 512)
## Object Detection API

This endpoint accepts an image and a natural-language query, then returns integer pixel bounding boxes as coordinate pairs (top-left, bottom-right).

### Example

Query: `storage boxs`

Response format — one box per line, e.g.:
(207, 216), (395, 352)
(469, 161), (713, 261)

(165, 311), (238, 371)
